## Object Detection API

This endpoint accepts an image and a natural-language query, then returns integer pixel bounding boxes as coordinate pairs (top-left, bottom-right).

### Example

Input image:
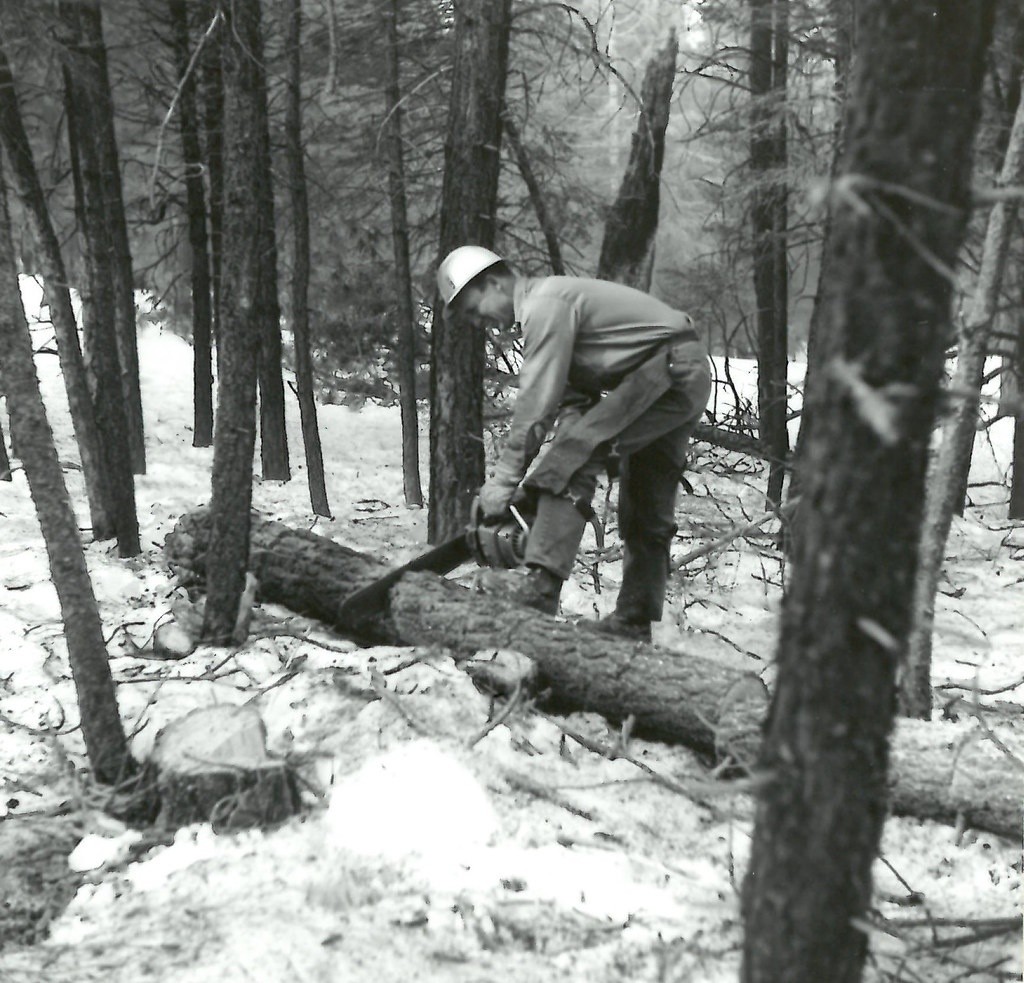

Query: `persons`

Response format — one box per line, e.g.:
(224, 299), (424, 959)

(434, 242), (713, 649)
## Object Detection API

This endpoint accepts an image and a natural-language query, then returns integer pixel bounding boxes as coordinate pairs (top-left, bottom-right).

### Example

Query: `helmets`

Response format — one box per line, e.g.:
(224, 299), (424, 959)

(435, 245), (507, 306)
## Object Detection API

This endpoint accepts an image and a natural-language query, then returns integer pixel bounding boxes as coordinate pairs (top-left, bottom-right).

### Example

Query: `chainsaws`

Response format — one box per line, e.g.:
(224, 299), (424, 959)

(337, 476), (544, 627)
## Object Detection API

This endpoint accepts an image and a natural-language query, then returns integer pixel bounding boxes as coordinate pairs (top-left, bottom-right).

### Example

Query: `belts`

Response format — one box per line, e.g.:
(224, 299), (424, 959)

(636, 324), (705, 366)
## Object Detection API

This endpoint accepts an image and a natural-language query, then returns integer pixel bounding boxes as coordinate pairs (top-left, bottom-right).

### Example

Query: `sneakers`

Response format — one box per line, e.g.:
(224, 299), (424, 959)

(479, 556), (562, 619)
(575, 607), (654, 643)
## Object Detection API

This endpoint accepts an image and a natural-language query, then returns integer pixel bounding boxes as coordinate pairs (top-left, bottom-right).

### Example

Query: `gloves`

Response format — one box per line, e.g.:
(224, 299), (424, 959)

(480, 467), (520, 517)
